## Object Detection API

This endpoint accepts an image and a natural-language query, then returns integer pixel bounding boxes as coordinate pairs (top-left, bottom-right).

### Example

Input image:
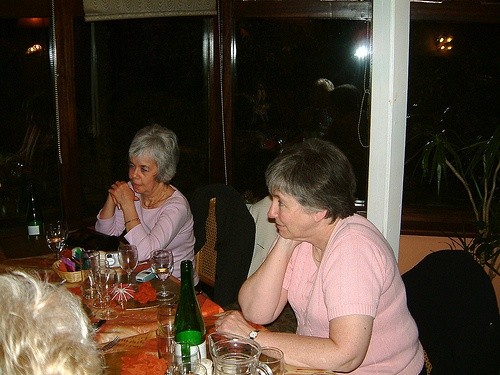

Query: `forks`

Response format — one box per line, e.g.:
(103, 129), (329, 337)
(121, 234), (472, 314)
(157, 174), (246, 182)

(101, 337), (120, 352)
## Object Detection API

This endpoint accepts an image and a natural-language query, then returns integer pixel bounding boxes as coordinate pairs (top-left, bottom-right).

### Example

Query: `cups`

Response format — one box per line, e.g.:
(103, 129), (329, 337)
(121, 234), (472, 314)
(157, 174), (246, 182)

(168, 342), (206, 375)
(252, 347), (284, 375)
(155, 324), (173, 360)
(80, 250), (100, 299)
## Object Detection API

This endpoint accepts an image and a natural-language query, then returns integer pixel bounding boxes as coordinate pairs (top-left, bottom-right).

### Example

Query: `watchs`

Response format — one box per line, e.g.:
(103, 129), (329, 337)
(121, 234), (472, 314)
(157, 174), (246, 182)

(249, 329), (261, 339)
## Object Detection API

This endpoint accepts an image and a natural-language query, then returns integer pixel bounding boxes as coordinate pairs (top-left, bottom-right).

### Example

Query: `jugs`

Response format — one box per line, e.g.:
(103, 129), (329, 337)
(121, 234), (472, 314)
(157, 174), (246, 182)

(207, 333), (273, 375)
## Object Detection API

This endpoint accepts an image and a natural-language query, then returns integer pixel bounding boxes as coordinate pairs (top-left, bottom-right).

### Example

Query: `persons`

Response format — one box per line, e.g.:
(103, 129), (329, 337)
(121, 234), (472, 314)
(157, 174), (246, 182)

(95, 124), (200, 291)
(215, 138), (429, 375)
(0, 268), (109, 375)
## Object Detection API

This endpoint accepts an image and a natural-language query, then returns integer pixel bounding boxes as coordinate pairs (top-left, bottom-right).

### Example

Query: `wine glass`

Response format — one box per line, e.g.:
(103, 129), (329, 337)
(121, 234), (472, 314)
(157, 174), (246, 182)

(117, 245), (139, 290)
(149, 249), (177, 301)
(96, 269), (119, 320)
(46, 229), (64, 261)
(89, 259), (111, 309)
(156, 305), (176, 364)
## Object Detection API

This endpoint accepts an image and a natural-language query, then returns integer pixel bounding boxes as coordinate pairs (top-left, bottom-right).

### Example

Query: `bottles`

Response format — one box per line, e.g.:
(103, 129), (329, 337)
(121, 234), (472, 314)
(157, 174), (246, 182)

(26, 183), (45, 240)
(173, 260), (206, 375)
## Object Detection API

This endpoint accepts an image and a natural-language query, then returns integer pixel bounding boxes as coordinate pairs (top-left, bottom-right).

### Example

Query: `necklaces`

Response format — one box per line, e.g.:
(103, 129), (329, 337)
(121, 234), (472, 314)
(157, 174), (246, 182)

(311, 246), (320, 263)
(142, 184), (168, 207)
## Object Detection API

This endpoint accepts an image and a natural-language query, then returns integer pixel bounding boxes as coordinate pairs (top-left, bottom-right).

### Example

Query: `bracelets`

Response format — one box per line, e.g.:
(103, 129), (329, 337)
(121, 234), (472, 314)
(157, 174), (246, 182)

(124, 217), (139, 225)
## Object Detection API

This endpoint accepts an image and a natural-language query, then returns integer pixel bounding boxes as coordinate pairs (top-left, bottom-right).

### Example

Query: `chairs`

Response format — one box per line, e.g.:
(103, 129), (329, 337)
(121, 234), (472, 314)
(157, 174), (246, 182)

(189, 183), (255, 304)
(400, 249), (500, 375)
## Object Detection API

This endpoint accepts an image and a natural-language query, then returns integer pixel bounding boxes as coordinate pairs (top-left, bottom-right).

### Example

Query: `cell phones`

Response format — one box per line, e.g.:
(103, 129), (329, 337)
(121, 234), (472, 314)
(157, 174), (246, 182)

(136, 269), (154, 282)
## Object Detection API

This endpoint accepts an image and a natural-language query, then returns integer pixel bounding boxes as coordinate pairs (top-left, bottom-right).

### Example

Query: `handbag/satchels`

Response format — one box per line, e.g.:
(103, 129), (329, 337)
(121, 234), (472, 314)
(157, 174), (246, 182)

(61, 227), (130, 253)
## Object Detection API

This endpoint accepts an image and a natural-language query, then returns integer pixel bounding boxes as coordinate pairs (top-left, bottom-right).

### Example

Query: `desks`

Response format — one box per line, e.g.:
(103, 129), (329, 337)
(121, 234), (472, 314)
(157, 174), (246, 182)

(0, 252), (278, 375)
